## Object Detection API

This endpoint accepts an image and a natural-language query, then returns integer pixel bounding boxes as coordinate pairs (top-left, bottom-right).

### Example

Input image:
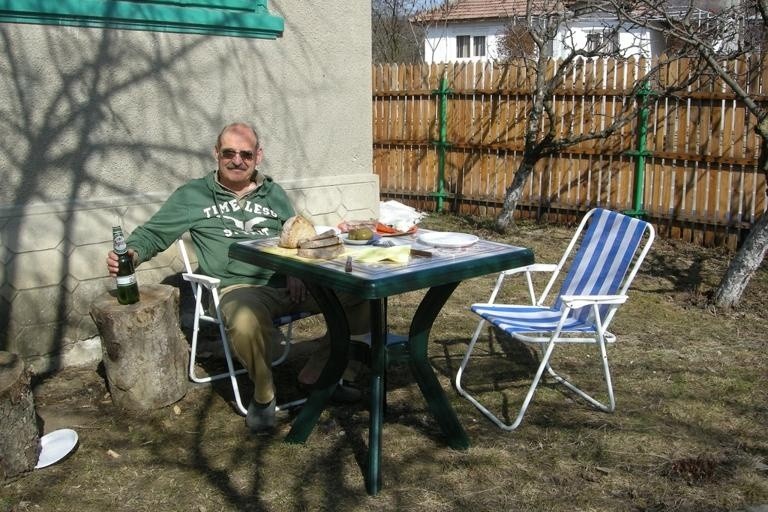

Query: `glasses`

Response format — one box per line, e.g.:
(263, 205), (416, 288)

(218, 147), (257, 161)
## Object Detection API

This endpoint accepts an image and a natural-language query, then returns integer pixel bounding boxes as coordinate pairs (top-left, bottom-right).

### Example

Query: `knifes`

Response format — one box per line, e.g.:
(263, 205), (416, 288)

(345, 255), (352, 272)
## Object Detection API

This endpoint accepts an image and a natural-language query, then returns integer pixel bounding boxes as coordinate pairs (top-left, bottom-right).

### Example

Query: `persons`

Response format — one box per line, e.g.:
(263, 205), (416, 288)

(106, 122), (371, 437)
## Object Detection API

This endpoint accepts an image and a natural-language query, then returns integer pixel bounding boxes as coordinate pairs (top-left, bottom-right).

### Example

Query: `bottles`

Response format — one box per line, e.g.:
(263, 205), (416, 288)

(112, 226), (141, 305)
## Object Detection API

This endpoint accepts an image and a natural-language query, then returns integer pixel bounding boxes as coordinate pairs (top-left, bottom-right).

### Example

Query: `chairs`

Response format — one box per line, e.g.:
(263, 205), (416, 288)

(456, 205), (656, 430)
(177, 231), (346, 415)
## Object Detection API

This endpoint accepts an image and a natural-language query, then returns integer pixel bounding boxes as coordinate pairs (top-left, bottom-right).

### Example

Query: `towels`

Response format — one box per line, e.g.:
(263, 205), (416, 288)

(354, 244), (411, 264)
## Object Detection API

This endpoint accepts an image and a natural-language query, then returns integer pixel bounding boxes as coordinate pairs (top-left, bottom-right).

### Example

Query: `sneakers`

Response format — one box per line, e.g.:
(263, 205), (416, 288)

(245, 393), (277, 433)
(295, 380), (363, 405)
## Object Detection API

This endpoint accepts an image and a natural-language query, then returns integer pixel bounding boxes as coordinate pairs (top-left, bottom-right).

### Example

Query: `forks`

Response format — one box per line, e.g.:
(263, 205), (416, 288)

(383, 241), (389, 248)
(388, 241), (433, 257)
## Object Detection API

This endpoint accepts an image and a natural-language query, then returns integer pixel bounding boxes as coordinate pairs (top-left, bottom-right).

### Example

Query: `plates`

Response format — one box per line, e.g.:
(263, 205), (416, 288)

(315, 225), (341, 238)
(34, 428), (78, 469)
(380, 231), (408, 237)
(419, 231), (479, 248)
(343, 237), (376, 245)
